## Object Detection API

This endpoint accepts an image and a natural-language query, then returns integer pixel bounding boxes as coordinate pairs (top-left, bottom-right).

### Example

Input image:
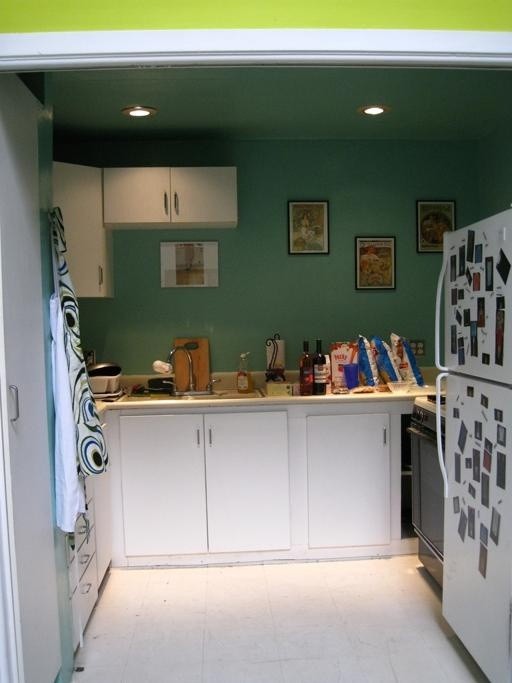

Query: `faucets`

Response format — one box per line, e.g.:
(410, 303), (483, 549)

(166, 345), (195, 392)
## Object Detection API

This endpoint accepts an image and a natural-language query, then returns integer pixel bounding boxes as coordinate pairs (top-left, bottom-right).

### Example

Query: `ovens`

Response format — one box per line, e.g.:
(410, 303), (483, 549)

(406, 404), (446, 565)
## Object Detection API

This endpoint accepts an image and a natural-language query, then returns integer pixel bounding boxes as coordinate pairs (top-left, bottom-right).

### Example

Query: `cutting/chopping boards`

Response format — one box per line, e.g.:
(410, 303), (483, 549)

(174, 337), (211, 392)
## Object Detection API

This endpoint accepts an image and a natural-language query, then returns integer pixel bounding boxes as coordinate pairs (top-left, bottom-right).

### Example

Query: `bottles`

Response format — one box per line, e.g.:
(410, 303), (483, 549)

(299, 338), (314, 396)
(313, 338), (327, 396)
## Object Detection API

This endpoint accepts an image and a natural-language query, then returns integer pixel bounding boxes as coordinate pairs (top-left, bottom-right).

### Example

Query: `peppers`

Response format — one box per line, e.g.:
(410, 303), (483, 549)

(133, 384), (145, 394)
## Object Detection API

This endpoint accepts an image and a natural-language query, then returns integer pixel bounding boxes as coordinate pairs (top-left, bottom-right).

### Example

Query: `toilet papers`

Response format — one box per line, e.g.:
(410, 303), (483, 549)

(267, 339), (285, 368)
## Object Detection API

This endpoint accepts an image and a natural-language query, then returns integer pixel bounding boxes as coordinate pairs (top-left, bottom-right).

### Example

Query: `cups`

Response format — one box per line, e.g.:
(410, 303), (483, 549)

(343, 363), (359, 390)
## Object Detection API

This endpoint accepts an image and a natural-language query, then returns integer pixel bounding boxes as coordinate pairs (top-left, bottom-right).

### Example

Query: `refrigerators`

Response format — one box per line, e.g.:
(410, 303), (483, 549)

(435, 207), (512, 683)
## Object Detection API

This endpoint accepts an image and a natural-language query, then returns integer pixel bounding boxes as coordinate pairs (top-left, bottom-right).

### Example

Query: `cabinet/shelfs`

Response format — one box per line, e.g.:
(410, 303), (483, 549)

(415, 199), (456, 253)
(53, 160), (114, 299)
(117, 403), (298, 567)
(102, 165), (239, 231)
(354, 234), (396, 290)
(299, 398), (414, 561)
(286, 198), (329, 255)
(65, 408), (112, 656)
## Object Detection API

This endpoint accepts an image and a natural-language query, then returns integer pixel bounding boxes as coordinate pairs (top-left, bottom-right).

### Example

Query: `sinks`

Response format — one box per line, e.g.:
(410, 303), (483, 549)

(191, 395), (260, 399)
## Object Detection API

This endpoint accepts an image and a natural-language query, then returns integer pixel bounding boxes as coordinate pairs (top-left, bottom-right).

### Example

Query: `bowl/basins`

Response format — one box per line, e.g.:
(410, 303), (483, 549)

(386, 380), (411, 395)
